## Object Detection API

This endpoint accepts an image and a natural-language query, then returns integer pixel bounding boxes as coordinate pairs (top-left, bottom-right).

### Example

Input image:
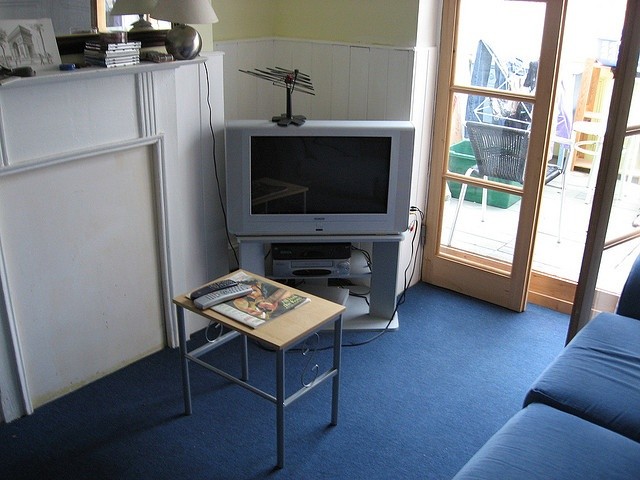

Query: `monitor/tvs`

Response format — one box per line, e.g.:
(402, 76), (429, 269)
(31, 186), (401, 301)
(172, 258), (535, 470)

(221, 117), (417, 239)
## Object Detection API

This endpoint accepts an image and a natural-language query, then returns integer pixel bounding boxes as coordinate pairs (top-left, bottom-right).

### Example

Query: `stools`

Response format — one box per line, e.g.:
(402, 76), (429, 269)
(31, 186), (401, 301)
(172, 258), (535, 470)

(560, 120), (634, 204)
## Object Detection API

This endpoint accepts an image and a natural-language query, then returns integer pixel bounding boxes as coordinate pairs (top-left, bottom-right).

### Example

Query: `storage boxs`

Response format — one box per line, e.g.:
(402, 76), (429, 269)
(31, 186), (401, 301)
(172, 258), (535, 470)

(449, 138), (521, 209)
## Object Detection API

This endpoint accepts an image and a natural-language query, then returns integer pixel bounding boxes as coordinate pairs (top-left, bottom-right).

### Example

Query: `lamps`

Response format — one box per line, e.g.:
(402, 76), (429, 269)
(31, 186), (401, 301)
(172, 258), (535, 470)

(151, 0), (219, 60)
(109, 0), (158, 31)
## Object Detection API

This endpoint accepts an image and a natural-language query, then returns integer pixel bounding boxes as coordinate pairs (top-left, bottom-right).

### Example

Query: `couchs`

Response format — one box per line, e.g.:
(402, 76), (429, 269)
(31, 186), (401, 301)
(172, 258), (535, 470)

(448, 252), (640, 479)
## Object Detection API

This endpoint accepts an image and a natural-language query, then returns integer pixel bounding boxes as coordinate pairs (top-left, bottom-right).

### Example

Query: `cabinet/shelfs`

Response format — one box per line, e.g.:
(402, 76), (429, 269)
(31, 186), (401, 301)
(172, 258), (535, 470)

(236, 234), (405, 331)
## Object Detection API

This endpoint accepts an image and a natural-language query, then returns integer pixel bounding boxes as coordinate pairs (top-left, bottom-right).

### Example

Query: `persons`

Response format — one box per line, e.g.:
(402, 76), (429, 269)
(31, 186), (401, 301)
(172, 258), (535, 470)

(13, 52), (54, 67)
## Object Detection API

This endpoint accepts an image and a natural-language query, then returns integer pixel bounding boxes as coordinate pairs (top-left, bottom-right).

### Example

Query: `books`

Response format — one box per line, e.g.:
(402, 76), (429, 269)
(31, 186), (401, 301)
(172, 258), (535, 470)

(185, 270), (310, 329)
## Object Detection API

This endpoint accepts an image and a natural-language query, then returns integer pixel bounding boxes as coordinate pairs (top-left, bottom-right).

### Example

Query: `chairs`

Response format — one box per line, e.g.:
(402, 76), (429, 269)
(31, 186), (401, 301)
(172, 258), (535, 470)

(448, 121), (566, 250)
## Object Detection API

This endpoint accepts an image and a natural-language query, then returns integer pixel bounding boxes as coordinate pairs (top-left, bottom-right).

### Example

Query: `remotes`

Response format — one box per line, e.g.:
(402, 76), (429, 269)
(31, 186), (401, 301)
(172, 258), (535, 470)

(189, 278), (238, 301)
(193, 282), (253, 310)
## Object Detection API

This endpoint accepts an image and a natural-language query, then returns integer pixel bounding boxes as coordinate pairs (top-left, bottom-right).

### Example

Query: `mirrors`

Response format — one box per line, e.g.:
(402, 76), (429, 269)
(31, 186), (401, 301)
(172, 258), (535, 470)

(96, 0), (172, 33)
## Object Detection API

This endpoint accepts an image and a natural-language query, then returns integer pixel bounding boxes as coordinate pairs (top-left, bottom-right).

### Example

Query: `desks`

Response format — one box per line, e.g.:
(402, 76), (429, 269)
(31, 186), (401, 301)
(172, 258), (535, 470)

(174, 267), (348, 467)
